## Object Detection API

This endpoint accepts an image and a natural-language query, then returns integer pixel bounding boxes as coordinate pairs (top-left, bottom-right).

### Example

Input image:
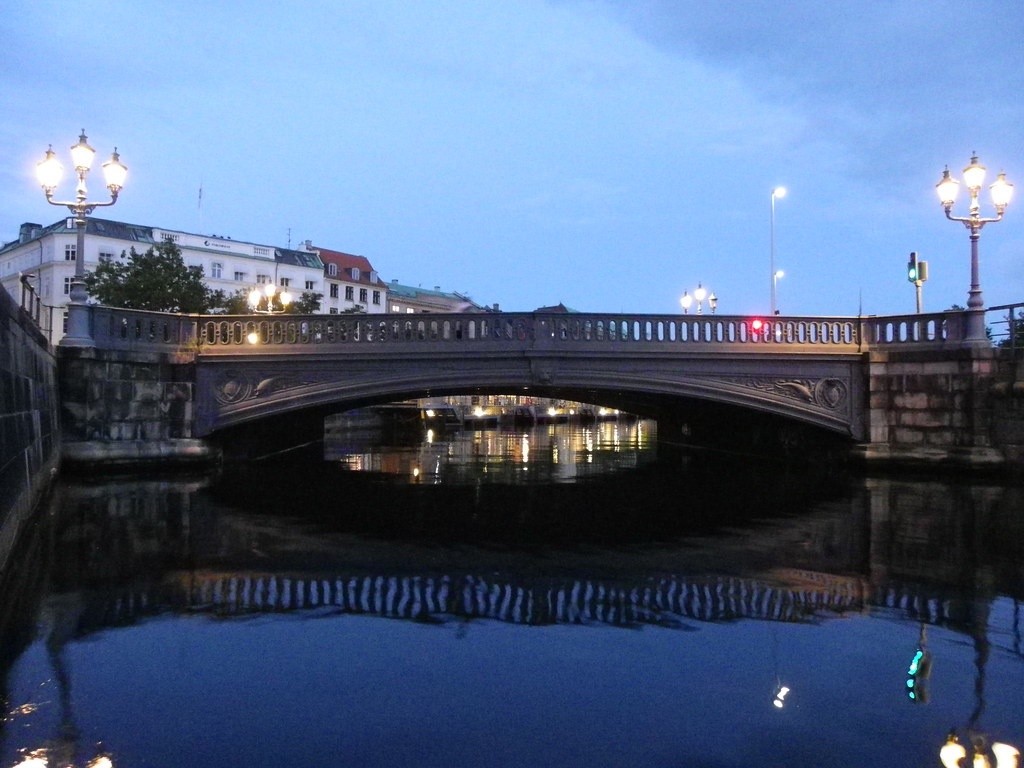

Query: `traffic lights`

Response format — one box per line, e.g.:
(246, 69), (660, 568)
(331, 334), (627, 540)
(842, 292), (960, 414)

(907, 252), (918, 282)
(904, 650), (929, 707)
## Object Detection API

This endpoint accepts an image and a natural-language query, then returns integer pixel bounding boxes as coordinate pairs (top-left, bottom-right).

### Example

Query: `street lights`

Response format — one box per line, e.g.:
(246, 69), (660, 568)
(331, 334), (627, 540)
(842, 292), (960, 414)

(773, 270), (784, 315)
(36, 128), (130, 348)
(933, 150), (1018, 344)
(770, 185), (787, 316)
(939, 633), (1020, 768)
